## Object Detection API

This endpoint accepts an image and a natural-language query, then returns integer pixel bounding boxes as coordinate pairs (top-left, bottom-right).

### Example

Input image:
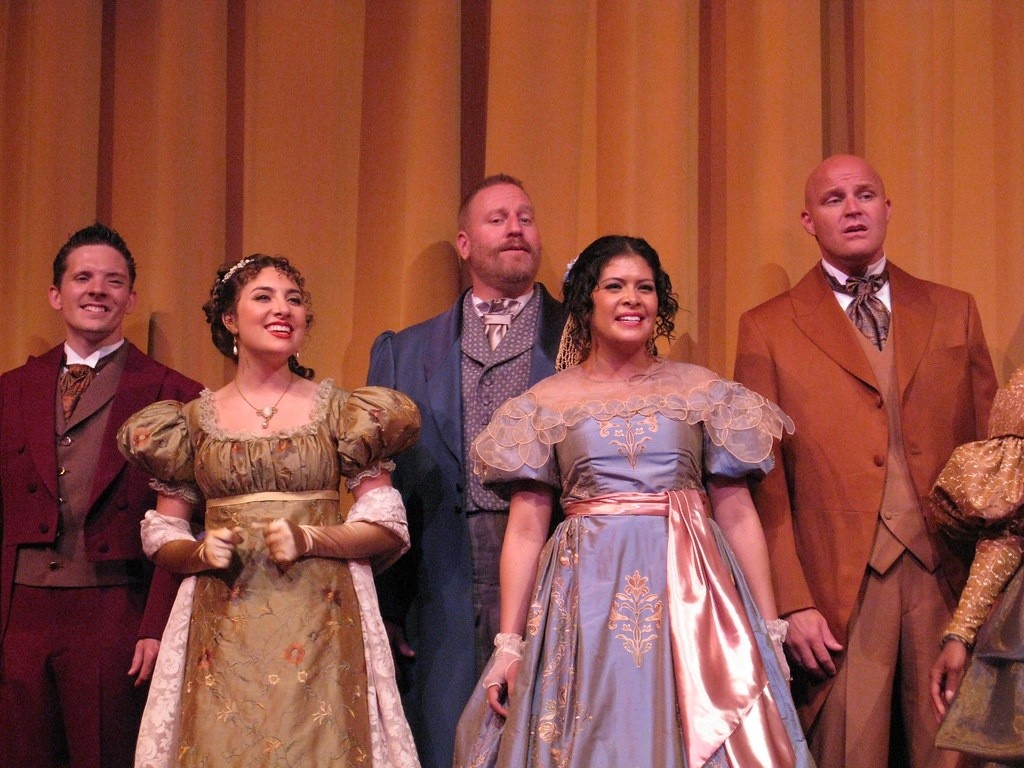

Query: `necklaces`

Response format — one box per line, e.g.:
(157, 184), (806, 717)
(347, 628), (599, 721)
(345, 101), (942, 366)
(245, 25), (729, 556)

(234, 370), (294, 428)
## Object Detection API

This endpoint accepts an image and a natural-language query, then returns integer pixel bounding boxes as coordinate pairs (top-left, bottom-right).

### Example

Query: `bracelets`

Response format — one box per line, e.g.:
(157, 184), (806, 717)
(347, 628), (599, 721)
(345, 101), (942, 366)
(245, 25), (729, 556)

(940, 635), (971, 652)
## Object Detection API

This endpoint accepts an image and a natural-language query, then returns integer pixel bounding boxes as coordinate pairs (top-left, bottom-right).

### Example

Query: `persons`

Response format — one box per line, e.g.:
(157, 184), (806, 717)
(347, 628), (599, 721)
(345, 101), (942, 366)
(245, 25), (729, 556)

(0, 222), (206, 768)
(731, 155), (998, 768)
(116, 253), (421, 768)
(366, 173), (570, 768)
(454, 235), (816, 768)
(928, 361), (1024, 768)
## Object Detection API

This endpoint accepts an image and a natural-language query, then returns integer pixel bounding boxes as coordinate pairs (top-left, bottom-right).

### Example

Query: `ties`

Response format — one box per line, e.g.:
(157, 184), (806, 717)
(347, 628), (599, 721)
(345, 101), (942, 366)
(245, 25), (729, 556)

(60, 348), (120, 426)
(823, 265), (891, 351)
(476, 297), (521, 351)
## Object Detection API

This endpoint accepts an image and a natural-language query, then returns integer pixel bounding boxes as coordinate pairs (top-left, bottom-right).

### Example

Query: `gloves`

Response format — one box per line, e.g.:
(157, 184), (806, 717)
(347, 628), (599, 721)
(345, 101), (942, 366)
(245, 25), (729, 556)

(251, 519), (403, 566)
(151, 527), (244, 575)
